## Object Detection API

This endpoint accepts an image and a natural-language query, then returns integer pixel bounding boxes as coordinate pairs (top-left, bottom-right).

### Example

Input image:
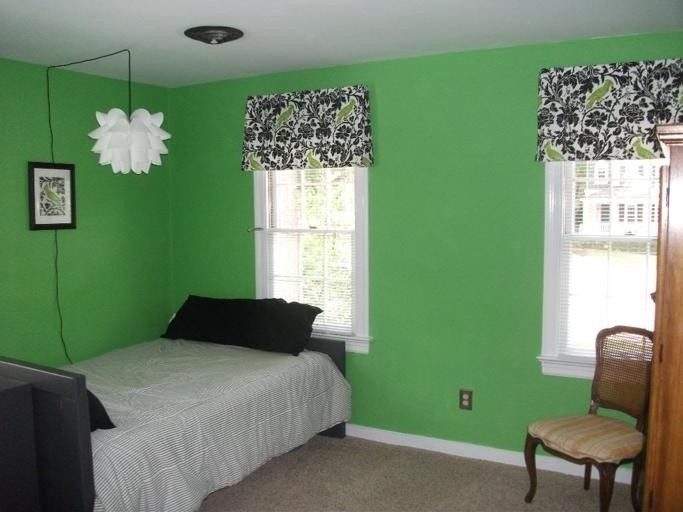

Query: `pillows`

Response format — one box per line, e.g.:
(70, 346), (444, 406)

(164, 292), (325, 355)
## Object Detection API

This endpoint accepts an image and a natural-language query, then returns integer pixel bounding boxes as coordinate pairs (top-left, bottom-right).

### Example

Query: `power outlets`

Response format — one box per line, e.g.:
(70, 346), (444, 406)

(457, 388), (473, 410)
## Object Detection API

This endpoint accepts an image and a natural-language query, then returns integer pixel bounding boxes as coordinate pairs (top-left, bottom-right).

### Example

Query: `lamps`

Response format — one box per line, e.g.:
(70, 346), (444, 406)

(44, 50), (171, 177)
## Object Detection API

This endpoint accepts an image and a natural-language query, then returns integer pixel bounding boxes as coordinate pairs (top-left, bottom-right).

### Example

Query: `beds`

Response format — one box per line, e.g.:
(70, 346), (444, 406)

(53, 336), (352, 511)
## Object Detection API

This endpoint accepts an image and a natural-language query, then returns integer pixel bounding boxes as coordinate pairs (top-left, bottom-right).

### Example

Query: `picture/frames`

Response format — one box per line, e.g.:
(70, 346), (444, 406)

(28, 162), (75, 230)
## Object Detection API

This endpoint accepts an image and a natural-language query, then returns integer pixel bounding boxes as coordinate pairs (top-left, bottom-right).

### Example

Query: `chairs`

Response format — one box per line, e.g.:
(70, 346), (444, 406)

(524, 326), (655, 512)
